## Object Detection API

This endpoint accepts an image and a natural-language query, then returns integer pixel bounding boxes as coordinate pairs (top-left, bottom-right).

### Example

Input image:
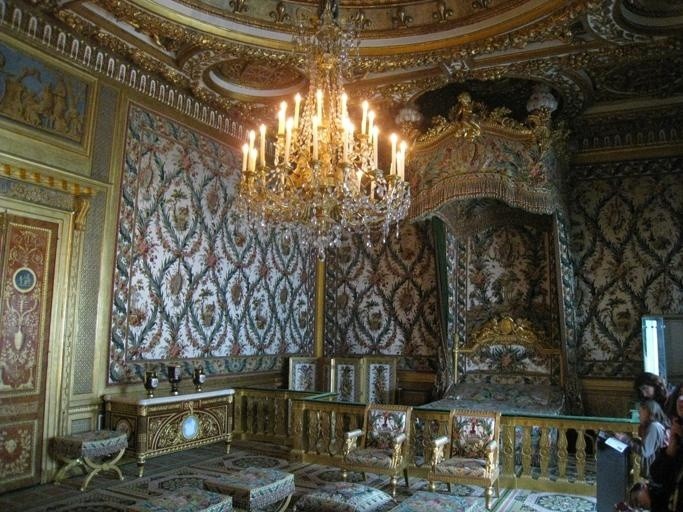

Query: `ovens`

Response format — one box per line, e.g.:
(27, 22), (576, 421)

(409, 311), (571, 467)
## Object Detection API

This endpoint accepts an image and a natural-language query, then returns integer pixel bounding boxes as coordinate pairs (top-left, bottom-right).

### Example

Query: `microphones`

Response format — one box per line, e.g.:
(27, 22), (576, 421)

(47, 428), (131, 493)
(386, 488), (481, 511)
(299, 480), (390, 512)
(137, 486), (236, 510)
(198, 463), (298, 511)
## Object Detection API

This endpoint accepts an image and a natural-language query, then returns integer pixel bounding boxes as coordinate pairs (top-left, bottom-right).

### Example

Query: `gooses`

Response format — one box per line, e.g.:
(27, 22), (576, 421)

(165, 363), (183, 396)
(191, 366), (206, 391)
(142, 369), (158, 397)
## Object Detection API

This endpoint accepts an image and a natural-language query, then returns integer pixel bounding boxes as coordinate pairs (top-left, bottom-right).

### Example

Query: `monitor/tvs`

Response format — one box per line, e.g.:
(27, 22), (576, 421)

(603, 436), (629, 455)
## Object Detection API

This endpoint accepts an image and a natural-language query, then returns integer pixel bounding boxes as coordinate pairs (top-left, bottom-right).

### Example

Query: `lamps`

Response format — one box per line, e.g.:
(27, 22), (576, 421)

(333, 399), (417, 503)
(422, 404), (506, 512)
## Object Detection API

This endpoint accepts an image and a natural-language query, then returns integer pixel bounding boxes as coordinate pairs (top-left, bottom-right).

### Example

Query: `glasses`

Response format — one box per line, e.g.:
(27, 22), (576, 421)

(237, 1), (413, 261)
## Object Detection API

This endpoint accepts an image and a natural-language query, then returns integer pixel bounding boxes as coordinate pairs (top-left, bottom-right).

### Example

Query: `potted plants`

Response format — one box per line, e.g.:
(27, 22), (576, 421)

(285, 355), (317, 395)
(331, 353), (364, 403)
(320, 356), (333, 403)
(364, 357), (398, 407)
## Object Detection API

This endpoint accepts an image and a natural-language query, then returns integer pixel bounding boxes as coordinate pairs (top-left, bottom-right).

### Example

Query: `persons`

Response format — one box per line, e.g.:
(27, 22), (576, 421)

(453, 91), (479, 123)
(612, 399), (671, 481)
(632, 372), (677, 416)
(629, 382), (683, 511)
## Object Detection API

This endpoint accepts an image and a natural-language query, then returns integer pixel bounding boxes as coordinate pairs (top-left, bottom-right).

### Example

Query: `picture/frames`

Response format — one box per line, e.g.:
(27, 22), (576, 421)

(101, 385), (239, 477)
